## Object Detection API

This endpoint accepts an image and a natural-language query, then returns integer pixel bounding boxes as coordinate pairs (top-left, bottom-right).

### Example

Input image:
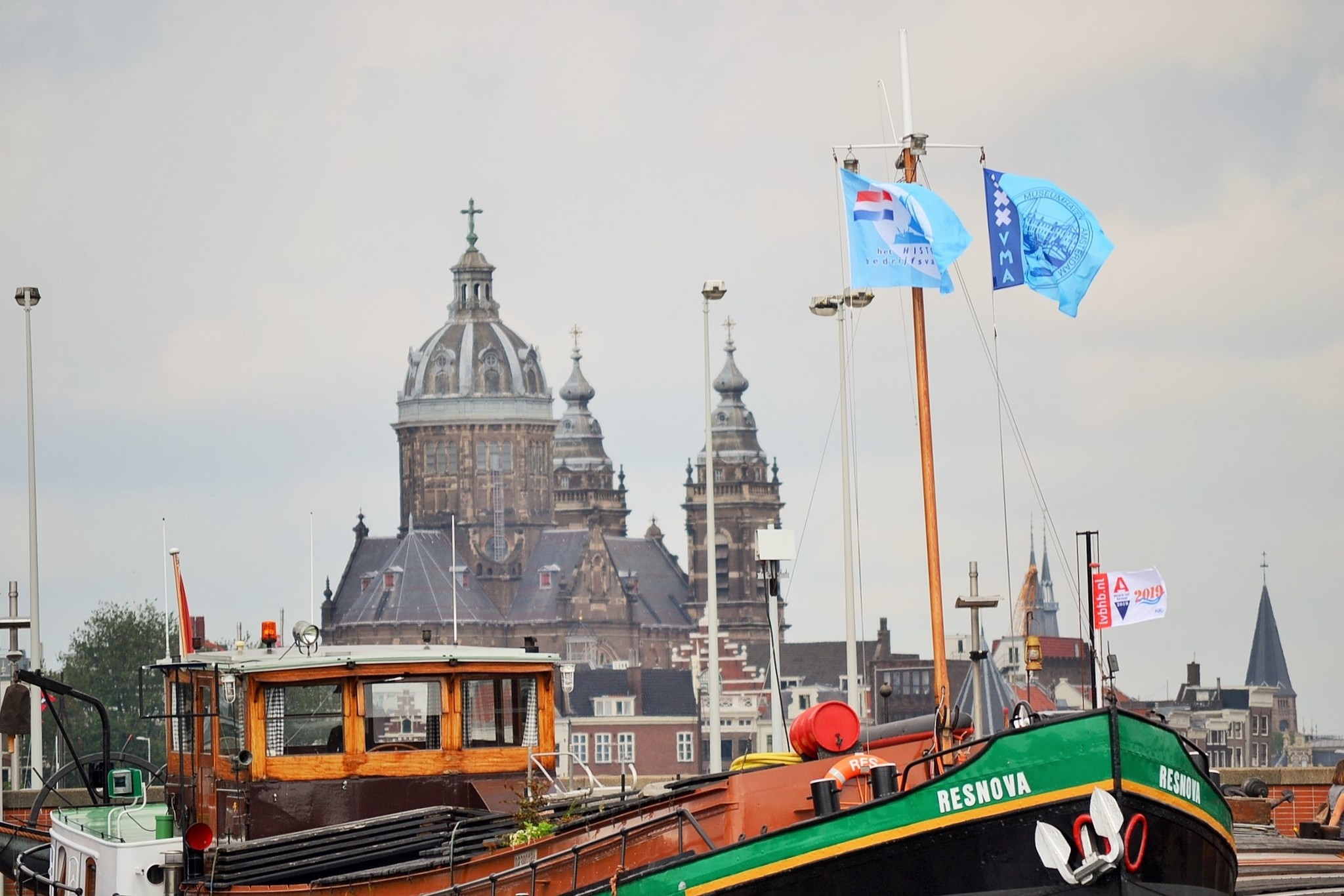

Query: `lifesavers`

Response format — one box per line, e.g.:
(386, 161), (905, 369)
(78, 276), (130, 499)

(824, 755), (909, 792)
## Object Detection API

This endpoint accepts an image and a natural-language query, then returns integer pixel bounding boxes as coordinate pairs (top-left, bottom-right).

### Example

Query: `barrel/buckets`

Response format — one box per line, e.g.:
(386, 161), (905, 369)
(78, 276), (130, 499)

(789, 700), (861, 759)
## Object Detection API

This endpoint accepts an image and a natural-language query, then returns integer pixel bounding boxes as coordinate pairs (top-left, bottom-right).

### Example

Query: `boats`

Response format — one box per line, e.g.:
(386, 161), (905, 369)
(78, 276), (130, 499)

(0, 24), (1239, 896)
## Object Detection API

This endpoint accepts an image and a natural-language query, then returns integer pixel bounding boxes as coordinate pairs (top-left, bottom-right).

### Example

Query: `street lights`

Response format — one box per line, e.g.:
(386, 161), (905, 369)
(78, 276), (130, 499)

(136, 736), (151, 786)
(14, 287), (42, 789)
(1023, 611), (1043, 705)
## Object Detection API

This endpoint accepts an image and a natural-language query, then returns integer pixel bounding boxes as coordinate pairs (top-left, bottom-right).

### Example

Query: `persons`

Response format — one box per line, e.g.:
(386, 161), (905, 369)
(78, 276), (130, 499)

(1327, 760), (1344, 838)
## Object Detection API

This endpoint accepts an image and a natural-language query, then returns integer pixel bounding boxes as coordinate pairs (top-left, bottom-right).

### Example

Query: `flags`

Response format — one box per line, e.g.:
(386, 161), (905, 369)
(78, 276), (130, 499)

(1092, 567), (1168, 630)
(983, 166), (1115, 317)
(839, 167), (973, 295)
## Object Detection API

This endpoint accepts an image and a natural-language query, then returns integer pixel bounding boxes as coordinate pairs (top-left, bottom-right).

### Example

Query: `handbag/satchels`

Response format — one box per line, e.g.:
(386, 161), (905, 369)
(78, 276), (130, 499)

(1314, 795), (1331, 825)
